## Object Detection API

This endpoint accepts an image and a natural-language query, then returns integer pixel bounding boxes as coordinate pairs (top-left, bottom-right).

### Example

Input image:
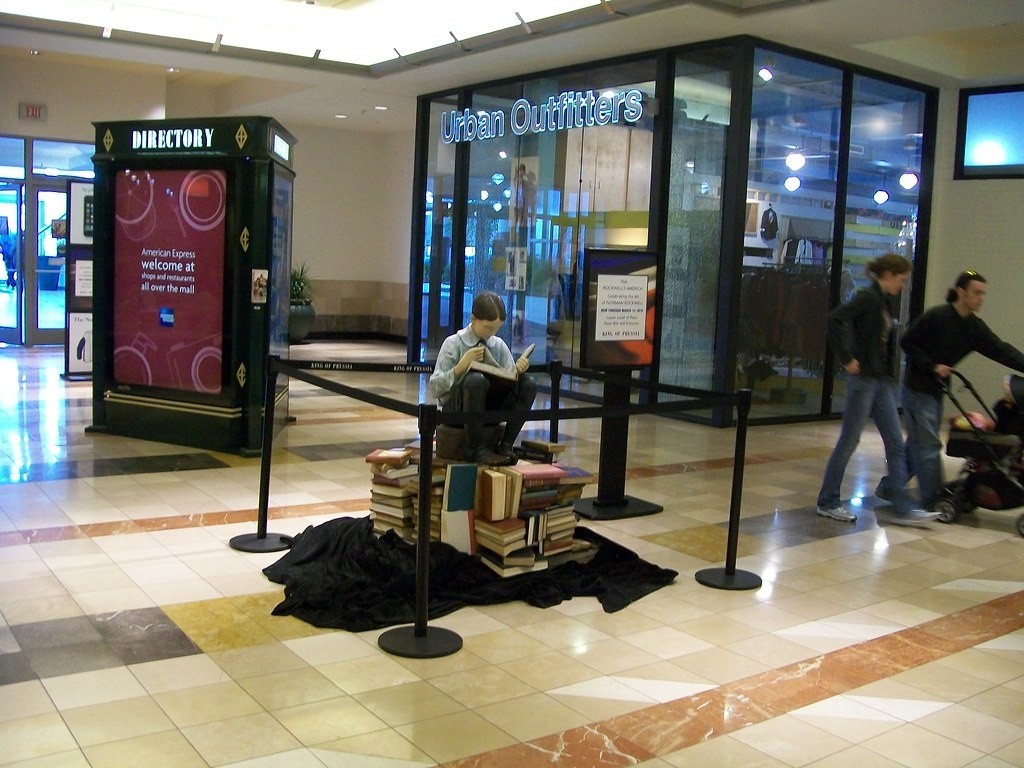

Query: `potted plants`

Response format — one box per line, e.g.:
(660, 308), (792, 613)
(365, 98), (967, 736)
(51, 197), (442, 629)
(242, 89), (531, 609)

(288, 260), (315, 344)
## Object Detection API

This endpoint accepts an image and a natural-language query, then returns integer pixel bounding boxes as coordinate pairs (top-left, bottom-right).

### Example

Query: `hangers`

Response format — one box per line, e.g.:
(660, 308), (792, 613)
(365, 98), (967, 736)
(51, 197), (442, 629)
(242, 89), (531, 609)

(749, 233), (851, 280)
(768, 203), (773, 212)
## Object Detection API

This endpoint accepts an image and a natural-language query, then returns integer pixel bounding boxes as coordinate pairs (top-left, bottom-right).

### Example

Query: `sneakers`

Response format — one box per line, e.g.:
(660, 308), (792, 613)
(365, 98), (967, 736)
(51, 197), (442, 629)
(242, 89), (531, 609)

(896, 510), (942, 524)
(816, 504), (857, 522)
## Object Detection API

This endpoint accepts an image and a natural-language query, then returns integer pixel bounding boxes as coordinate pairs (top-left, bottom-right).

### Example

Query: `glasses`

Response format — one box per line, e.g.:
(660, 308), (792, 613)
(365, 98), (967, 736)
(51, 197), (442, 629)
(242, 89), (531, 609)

(964, 270), (976, 277)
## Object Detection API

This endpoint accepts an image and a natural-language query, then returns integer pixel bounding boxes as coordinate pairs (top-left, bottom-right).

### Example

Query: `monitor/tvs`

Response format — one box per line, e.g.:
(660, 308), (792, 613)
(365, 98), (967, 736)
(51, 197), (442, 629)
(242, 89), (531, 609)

(954, 82), (1024, 180)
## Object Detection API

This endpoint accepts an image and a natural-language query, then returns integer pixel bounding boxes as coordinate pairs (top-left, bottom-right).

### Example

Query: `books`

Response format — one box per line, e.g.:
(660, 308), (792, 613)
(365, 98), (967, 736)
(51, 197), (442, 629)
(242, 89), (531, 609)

(466, 339), (536, 382)
(359, 438), (592, 577)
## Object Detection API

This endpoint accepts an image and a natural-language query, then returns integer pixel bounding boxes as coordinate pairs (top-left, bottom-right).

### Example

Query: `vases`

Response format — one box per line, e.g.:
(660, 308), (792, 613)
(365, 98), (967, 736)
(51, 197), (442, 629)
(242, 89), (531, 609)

(39, 255), (62, 290)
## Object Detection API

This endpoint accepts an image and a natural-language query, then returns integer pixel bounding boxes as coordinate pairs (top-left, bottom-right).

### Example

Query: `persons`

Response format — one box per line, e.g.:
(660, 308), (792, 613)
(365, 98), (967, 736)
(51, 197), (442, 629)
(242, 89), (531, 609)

(869, 269), (1024, 512)
(3, 232), (17, 290)
(429, 291), (538, 466)
(817, 253), (944, 525)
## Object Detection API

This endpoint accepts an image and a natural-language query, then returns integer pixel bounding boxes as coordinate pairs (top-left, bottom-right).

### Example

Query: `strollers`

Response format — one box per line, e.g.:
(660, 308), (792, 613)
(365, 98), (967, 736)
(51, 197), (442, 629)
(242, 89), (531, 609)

(933, 368), (1024, 537)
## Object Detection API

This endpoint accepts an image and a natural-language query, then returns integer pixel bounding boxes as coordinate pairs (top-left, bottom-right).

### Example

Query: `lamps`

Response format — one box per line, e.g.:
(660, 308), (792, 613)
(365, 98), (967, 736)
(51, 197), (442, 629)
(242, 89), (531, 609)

(874, 175), (890, 204)
(899, 153), (918, 189)
(785, 168), (801, 193)
(785, 128), (806, 172)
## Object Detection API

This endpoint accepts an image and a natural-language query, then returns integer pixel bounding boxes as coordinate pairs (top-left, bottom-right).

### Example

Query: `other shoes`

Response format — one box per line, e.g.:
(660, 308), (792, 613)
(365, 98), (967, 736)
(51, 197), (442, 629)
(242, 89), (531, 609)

(875, 478), (914, 502)
